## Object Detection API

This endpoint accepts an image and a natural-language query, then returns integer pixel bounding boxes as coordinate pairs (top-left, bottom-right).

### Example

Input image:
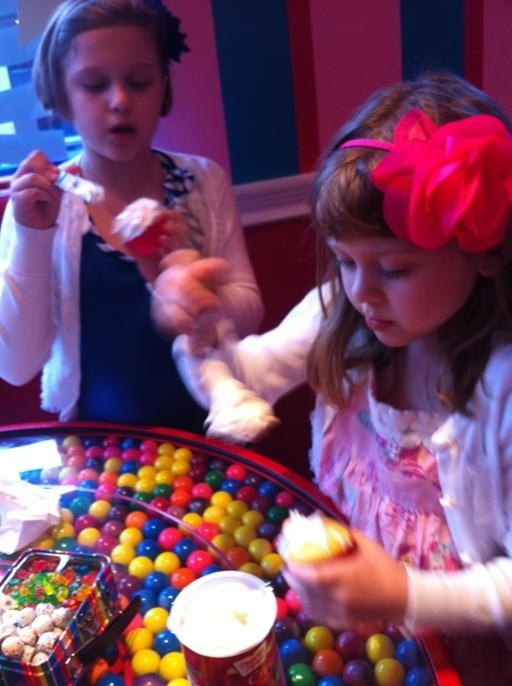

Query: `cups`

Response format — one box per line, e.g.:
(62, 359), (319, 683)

(168, 571), (289, 686)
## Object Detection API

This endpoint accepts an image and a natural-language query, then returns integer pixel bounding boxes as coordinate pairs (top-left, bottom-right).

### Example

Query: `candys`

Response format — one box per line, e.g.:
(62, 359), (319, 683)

(0, 433), (432, 686)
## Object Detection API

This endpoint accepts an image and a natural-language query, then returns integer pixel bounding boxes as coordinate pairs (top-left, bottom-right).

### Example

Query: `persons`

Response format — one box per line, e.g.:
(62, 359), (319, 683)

(1, 0), (265, 433)
(170, 71), (512, 643)
